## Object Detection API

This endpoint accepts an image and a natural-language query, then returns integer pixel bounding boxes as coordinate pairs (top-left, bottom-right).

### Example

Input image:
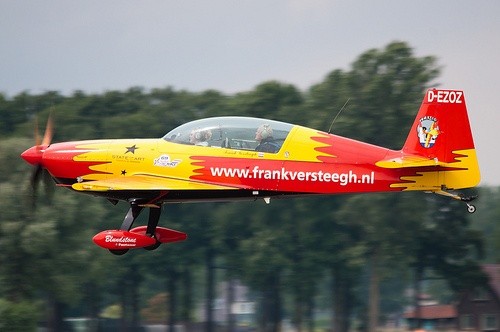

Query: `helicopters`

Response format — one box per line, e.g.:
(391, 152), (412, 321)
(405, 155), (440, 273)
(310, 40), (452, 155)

(19, 85), (483, 254)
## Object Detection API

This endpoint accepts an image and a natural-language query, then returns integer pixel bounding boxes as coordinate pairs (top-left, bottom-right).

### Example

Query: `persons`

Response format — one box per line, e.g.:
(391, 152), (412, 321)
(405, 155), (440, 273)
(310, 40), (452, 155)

(254, 124), (281, 153)
(174, 126), (212, 147)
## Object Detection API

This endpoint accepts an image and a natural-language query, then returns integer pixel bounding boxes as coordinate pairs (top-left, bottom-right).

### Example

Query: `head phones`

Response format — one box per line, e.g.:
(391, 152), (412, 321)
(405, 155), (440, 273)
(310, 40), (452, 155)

(195, 132), (204, 140)
(261, 124), (270, 140)
